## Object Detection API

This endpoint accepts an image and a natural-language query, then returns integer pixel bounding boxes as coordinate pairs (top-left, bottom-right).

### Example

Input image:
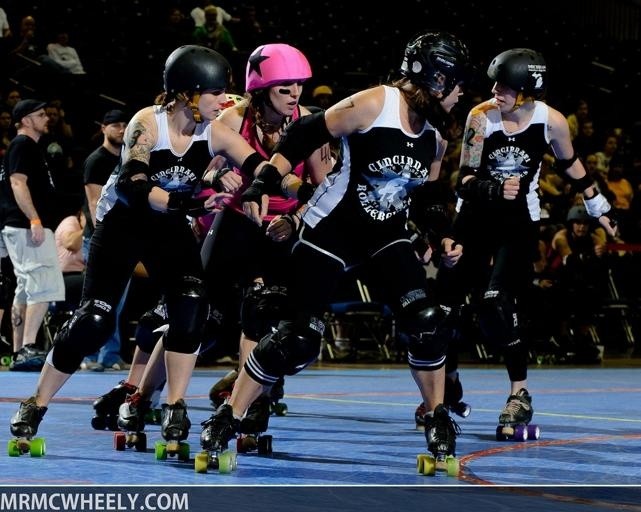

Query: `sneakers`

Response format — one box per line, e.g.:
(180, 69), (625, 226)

(25, 346), (48, 357)
(15, 343), (48, 372)
(11, 354), (44, 372)
(81, 360), (103, 372)
(105, 361), (130, 370)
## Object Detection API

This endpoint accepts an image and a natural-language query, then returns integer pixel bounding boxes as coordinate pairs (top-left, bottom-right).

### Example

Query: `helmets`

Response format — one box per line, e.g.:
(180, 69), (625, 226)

(400, 28), (477, 89)
(567, 205), (592, 226)
(163, 45), (234, 94)
(487, 48), (548, 94)
(245, 43), (313, 92)
(312, 85), (333, 97)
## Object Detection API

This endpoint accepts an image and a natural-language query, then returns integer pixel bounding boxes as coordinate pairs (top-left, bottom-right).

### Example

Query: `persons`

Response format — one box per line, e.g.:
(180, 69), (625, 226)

(0, 0), (641, 454)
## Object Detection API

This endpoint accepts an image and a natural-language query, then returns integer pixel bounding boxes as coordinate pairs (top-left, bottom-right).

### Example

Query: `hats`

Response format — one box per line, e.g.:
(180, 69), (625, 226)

(103, 109), (130, 126)
(13, 99), (47, 124)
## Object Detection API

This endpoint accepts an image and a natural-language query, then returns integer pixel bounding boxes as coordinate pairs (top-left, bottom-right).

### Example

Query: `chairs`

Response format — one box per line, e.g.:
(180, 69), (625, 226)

(29, 266), (640, 374)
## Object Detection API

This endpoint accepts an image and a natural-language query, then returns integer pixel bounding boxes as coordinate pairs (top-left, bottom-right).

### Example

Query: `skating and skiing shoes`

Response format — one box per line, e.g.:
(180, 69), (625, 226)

(7, 395), (49, 457)
(414, 372), (471, 432)
(496, 387), (540, 441)
(416, 405), (463, 477)
(91, 368), (288, 474)
